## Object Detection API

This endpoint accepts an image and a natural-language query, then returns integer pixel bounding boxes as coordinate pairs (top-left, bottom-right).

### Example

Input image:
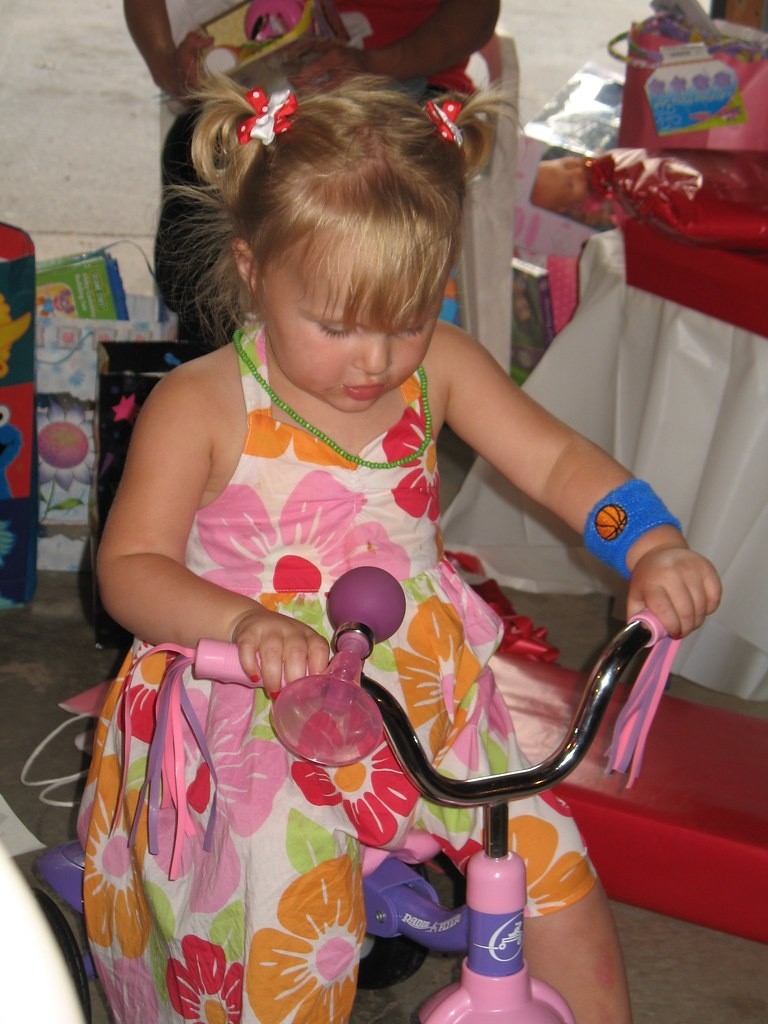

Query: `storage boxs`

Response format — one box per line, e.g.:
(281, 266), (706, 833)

(622, 216), (768, 338)
(511, 122), (612, 387)
(497, 653), (768, 943)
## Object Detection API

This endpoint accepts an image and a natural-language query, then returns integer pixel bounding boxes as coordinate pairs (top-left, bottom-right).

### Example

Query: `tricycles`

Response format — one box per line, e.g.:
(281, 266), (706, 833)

(26, 567), (684, 1023)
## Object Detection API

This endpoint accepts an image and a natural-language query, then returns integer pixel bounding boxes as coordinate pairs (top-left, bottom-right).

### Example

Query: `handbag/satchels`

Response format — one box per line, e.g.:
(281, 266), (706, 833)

(20, 679), (115, 804)
(608, 19), (768, 150)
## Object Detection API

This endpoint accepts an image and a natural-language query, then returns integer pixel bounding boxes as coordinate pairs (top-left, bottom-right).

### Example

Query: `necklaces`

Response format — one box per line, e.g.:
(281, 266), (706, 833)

(232, 329), (431, 469)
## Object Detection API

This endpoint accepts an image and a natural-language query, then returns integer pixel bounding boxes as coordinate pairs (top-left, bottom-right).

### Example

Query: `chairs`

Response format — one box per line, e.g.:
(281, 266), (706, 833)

(460, 34), (520, 377)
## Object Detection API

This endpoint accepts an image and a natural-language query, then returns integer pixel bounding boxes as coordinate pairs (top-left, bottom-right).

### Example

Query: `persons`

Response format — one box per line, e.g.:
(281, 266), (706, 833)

(78, 0), (722, 1024)
